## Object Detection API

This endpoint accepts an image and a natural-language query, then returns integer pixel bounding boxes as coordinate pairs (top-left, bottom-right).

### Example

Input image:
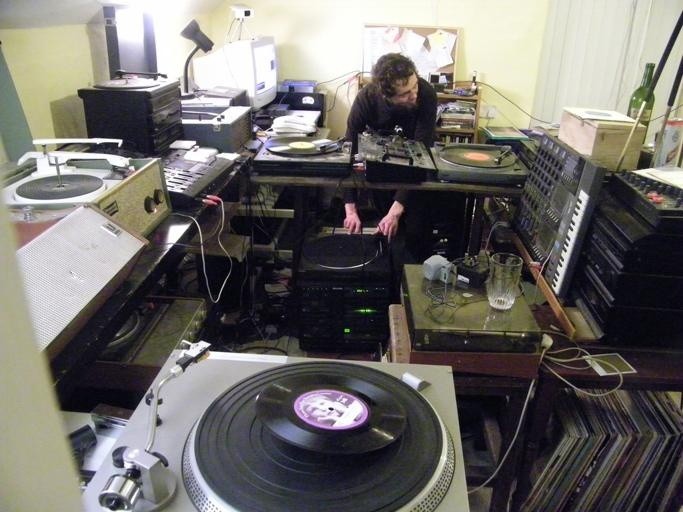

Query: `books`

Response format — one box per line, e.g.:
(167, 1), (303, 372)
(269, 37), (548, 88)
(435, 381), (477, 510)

(513, 387), (683, 512)
(478, 124), (528, 141)
(435, 98), (476, 144)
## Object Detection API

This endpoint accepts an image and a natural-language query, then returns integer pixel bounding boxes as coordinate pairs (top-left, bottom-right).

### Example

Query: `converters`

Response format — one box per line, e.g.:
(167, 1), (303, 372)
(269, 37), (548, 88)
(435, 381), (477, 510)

(457, 254), (490, 287)
(423, 253), (457, 285)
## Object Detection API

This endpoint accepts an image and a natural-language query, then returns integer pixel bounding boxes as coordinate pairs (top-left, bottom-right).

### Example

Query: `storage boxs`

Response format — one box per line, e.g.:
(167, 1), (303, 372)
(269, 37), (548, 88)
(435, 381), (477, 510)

(558, 111), (647, 170)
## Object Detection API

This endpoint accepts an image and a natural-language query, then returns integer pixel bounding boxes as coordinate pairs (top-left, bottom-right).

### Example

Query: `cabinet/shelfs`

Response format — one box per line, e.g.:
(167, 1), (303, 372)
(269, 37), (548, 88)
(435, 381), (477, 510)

(358, 75), (483, 144)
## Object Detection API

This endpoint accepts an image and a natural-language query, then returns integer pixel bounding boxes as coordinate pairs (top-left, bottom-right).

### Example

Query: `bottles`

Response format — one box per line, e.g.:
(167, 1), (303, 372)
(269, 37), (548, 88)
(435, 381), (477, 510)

(626, 62), (654, 144)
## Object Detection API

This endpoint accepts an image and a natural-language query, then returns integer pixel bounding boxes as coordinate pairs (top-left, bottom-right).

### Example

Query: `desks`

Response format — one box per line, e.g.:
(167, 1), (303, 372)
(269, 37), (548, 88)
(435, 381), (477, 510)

(52, 155), (250, 396)
(508, 304), (683, 511)
(250, 177), (525, 275)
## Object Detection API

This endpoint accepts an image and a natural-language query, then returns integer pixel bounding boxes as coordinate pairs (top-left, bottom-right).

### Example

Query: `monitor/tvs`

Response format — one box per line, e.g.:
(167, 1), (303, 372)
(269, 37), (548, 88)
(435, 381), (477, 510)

(189, 36), (278, 112)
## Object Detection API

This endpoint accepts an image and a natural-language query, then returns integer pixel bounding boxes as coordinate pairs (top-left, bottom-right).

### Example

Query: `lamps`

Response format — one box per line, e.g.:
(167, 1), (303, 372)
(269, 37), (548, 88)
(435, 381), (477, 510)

(180, 20), (214, 99)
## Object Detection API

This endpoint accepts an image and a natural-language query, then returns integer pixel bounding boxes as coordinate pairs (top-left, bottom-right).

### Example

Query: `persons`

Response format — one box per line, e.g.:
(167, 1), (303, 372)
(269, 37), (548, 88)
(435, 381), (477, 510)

(342, 50), (439, 245)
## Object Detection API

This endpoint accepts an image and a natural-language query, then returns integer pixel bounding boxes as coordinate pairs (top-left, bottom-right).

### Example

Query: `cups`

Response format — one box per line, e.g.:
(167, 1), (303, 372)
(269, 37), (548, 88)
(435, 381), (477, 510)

(486, 251), (524, 313)
